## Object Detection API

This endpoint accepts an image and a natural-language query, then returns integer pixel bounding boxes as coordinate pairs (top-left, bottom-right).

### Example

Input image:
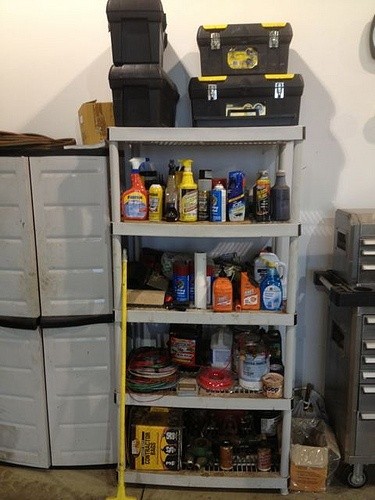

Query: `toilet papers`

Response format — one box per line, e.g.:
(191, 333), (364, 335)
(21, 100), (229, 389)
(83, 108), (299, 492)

(194, 252), (206, 309)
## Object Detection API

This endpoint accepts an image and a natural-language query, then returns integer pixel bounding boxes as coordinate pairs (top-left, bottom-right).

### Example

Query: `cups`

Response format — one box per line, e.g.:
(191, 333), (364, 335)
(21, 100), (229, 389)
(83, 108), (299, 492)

(262, 373), (284, 399)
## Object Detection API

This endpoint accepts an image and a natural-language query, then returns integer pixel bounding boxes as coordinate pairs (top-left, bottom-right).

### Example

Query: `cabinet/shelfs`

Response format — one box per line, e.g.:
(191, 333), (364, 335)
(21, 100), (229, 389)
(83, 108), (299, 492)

(107, 125), (306, 496)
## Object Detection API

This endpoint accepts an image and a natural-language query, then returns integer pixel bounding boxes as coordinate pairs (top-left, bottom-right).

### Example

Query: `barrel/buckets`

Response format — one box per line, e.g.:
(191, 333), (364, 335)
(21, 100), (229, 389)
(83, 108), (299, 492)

(238, 351), (272, 391)
(208, 325), (233, 369)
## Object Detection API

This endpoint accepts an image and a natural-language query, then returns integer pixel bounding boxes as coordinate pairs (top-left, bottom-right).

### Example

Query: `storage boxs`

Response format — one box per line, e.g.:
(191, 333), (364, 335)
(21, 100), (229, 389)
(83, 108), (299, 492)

(78, 0), (303, 146)
(127, 271), (169, 306)
(132, 408), (183, 470)
(290, 417), (340, 491)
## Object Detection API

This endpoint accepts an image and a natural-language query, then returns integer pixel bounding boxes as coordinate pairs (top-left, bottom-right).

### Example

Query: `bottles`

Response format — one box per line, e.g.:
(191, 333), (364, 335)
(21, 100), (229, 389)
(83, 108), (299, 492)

(254, 246), (287, 300)
(255, 169), (271, 222)
(168, 323), (202, 372)
(211, 180), (227, 222)
(259, 262), (283, 311)
(220, 440), (233, 470)
(271, 169), (291, 222)
(258, 439), (272, 471)
(172, 260), (213, 305)
(198, 170), (213, 221)
(148, 178), (163, 222)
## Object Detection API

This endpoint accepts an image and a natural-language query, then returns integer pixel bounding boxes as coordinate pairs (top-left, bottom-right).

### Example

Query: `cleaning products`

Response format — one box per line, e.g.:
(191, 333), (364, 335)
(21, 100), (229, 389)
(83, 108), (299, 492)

(175, 246), (286, 312)
(121, 156), (290, 222)
